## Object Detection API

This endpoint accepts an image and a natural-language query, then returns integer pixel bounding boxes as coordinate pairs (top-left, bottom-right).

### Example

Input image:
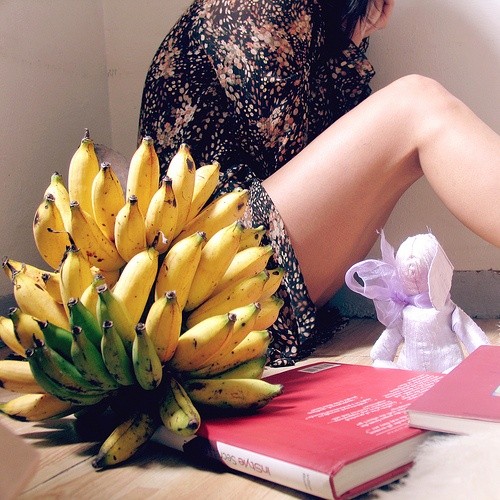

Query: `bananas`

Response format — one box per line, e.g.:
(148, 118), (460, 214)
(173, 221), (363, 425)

(0, 128), (288, 472)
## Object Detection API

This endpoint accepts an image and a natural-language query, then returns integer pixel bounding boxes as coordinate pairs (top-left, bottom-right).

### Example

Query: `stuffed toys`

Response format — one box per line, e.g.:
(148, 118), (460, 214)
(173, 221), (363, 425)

(344, 226), (491, 373)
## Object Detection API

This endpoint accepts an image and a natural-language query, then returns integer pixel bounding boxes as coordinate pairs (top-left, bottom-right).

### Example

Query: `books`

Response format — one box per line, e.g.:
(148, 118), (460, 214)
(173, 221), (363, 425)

(406, 344), (500, 435)
(150, 360), (445, 500)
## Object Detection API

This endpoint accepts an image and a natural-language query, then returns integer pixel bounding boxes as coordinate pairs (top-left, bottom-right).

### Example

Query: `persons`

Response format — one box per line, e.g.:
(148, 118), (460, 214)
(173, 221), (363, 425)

(135, 1), (500, 368)
